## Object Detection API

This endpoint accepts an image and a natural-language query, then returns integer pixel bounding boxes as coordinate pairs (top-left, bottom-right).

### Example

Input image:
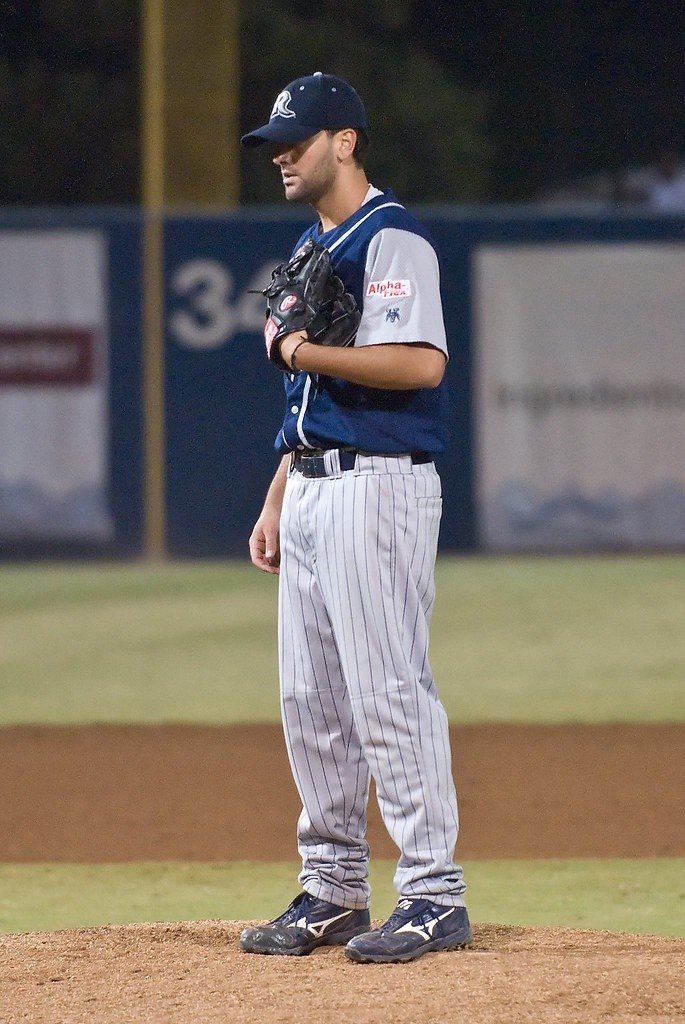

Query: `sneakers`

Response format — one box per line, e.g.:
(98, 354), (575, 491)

(240, 891), (370, 955)
(345, 899), (471, 963)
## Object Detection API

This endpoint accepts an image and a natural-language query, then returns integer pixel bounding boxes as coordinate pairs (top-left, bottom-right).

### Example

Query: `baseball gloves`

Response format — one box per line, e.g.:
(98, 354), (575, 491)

(243, 235), (363, 377)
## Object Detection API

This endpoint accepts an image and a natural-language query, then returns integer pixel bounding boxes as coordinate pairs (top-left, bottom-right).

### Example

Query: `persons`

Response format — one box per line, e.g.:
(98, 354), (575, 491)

(238, 71), (471, 965)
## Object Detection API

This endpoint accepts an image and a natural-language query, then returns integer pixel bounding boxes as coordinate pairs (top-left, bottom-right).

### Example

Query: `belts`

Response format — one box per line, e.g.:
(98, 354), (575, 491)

(294, 449), (432, 480)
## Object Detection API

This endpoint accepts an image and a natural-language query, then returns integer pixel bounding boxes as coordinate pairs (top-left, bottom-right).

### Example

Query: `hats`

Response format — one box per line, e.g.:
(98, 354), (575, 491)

(240, 71), (368, 144)
